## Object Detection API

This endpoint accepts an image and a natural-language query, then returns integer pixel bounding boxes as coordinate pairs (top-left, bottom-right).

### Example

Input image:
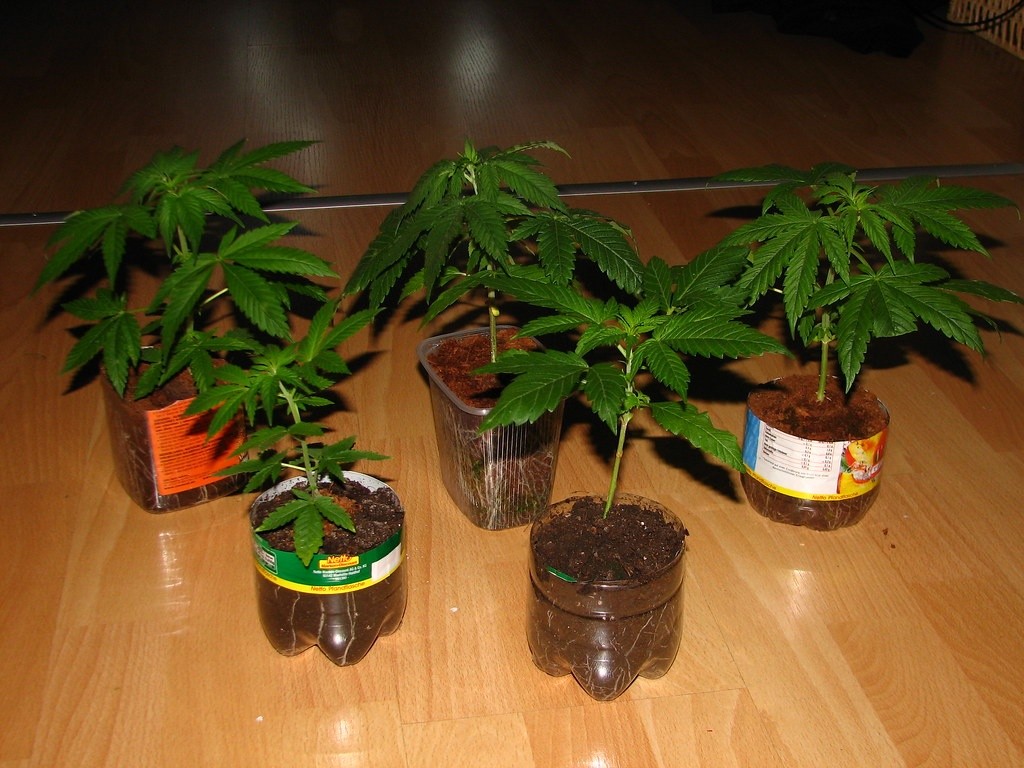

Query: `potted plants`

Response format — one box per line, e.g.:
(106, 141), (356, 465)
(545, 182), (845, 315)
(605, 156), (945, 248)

(26, 137), (321, 510)
(668, 159), (1024, 533)
(469, 242), (797, 703)
(340, 133), (647, 533)
(184, 292), (408, 665)
